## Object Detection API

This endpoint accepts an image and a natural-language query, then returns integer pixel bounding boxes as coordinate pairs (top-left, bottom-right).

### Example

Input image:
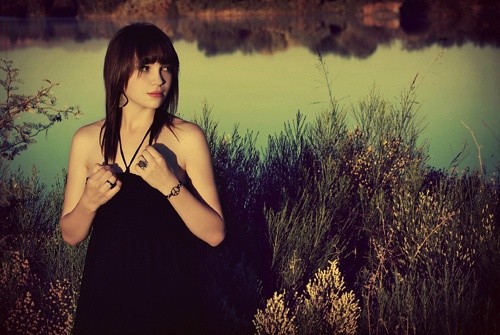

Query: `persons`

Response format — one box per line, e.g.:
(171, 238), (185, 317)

(58, 21), (228, 335)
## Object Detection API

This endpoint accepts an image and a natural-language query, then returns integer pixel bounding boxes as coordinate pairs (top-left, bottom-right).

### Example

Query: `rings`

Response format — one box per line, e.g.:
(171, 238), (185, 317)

(138, 161), (146, 169)
(106, 180), (114, 187)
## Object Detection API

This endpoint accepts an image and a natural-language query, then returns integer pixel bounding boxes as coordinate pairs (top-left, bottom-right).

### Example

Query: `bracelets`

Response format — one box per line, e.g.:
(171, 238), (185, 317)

(163, 180), (184, 199)
